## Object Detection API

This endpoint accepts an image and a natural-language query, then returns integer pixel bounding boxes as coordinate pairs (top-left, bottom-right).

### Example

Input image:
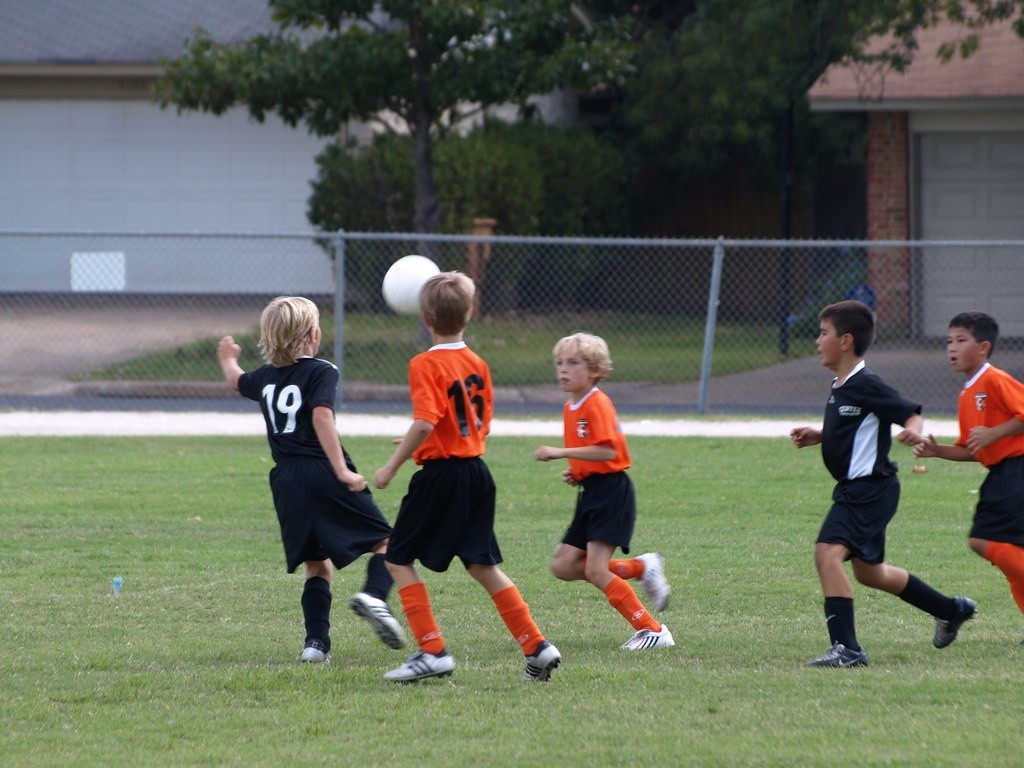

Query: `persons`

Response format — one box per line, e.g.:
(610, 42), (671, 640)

(535, 333), (676, 650)
(217, 295), (408, 662)
(790, 302), (978, 668)
(912, 311), (1024, 648)
(373, 271), (563, 683)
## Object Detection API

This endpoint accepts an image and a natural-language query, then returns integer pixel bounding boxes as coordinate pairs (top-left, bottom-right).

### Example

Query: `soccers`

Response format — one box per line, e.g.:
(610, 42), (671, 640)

(381, 255), (441, 318)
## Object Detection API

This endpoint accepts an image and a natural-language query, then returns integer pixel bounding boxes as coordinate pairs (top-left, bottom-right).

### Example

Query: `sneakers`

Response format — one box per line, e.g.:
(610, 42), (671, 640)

(352, 591), (406, 649)
(302, 642), (329, 662)
(933, 595), (980, 648)
(522, 639), (561, 681)
(635, 549), (671, 614)
(382, 649), (456, 683)
(808, 641), (870, 669)
(620, 623), (677, 652)
